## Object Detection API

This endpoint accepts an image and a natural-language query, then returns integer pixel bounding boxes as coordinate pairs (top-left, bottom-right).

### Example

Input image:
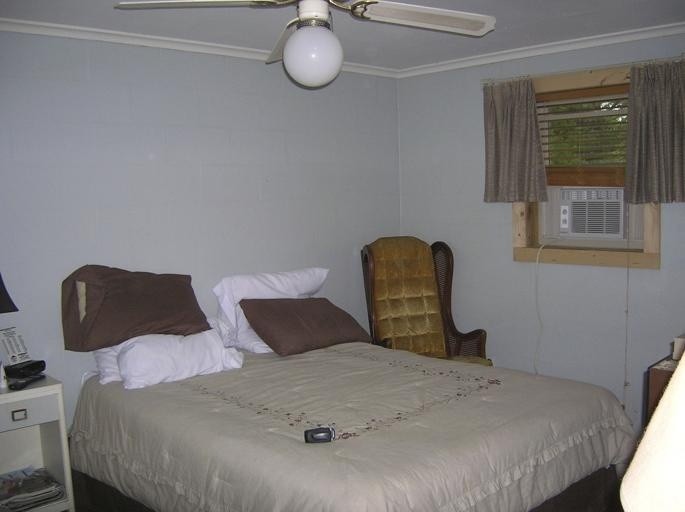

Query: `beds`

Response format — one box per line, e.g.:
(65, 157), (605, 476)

(68, 343), (637, 512)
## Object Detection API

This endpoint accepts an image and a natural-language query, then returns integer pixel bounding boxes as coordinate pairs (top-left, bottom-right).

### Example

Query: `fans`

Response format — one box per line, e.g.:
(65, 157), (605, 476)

(117, 0), (497, 64)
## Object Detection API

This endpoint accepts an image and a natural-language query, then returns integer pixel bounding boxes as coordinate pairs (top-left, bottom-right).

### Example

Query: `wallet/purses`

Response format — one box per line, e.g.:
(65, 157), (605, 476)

(4, 359), (45, 378)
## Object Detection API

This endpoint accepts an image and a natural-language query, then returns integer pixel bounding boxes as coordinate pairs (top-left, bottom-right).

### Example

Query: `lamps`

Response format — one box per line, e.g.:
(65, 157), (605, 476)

(0, 273), (20, 313)
(283, 0), (344, 87)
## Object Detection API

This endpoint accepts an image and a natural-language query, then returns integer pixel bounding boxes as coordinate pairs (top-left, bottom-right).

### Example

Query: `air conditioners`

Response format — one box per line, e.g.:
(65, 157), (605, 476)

(538, 186), (644, 251)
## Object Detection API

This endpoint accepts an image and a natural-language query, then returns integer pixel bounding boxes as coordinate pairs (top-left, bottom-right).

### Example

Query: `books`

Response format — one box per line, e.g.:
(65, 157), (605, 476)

(0, 467), (67, 512)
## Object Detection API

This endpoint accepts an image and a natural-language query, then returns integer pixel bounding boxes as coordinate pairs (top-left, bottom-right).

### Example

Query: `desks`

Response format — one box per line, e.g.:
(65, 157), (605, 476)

(647, 354), (679, 418)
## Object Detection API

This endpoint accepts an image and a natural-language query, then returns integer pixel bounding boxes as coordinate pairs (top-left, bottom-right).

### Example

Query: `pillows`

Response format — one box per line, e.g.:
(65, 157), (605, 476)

(62, 264), (212, 353)
(235, 306), (273, 355)
(116, 329), (245, 390)
(93, 342), (121, 385)
(212, 267), (330, 347)
(238, 298), (369, 357)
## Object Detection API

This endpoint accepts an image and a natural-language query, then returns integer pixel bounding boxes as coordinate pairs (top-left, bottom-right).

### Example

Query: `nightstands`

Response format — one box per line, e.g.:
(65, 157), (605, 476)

(0, 376), (75, 512)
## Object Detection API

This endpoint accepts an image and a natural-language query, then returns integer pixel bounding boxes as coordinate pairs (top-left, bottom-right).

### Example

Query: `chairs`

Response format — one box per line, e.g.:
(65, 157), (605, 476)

(362, 236), (493, 369)
